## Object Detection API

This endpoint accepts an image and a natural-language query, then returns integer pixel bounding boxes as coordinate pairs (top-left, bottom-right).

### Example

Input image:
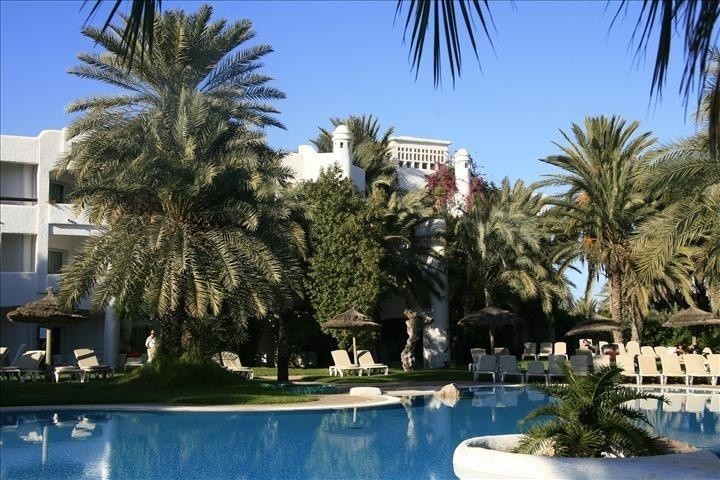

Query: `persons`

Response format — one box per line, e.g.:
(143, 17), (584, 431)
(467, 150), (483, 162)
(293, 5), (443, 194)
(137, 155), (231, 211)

(674, 342), (687, 371)
(144, 330), (157, 363)
(693, 342), (702, 354)
(581, 339), (596, 356)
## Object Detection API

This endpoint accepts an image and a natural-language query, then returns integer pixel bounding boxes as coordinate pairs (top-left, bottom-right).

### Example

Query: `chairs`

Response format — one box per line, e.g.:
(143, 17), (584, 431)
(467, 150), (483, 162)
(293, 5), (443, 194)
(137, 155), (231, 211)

(328, 349), (389, 378)
(124, 357), (143, 371)
(0, 411), (96, 442)
(211, 351), (254, 381)
(0, 347), (115, 383)
(618, 386), (720, 414)
(471, 387), (561, 407)
(470, 337), (719, 386)
